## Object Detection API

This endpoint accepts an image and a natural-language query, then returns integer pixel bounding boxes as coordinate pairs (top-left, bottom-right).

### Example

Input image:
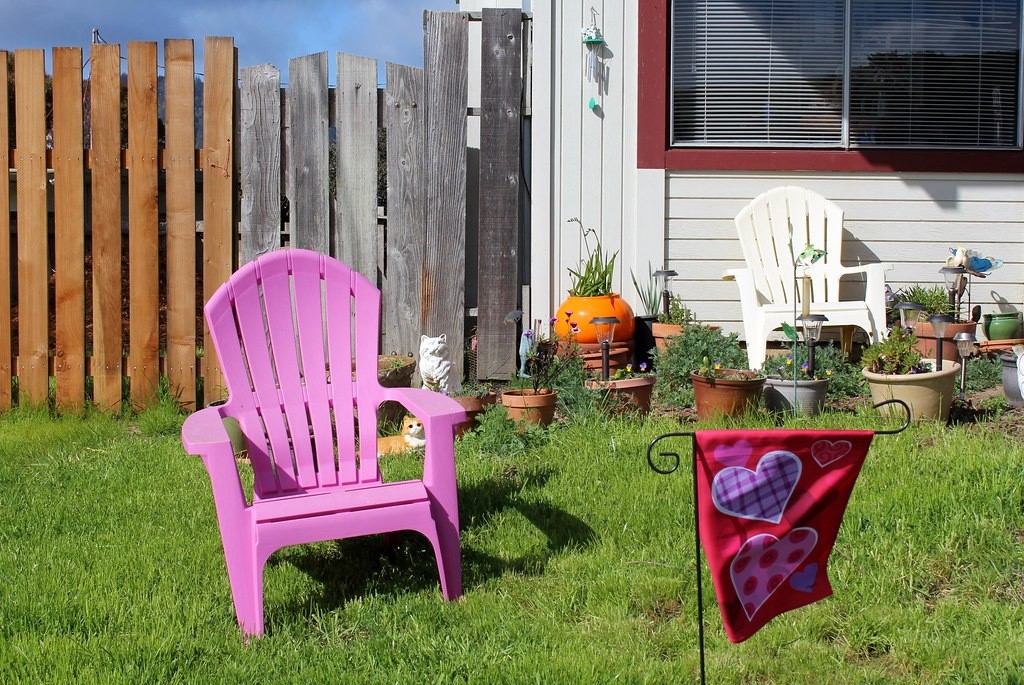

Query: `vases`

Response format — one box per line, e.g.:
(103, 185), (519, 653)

(584, 377), (654, 421)
(690, 368), (765, 421)
(1000, 354), (1024, 408)
(763, 374), (829, 416)
(981, 312), (1020, 342)
(862, 358), (965, 422)
(502, 389), (557, 431)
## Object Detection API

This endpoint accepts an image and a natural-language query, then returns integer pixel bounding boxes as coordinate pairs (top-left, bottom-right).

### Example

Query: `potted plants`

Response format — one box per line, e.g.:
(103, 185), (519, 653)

(628, 260), (663, 359)
(899, 283), (977, 362)
(445, 390), (481, 439)
(327, 353), (415, 425)
(208, 380), (247, 454)
(552, 218), (634, 344)
(652, 294), (720, 347)
(467, 382), (497, 405)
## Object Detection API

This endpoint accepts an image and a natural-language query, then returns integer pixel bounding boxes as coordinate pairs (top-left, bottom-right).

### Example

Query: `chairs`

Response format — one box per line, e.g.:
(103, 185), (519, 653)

(720, 185), (894, 373)
(181, 247), (467, 645)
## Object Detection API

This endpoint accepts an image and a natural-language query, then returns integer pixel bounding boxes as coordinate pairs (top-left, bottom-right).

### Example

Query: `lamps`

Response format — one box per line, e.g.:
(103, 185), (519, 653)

(938, 266), (967, 293)
(589, 316), (620, 350)
(796, 315), (829, 345)
(653, 270), (678, 297)
(953, 333), (978, 358)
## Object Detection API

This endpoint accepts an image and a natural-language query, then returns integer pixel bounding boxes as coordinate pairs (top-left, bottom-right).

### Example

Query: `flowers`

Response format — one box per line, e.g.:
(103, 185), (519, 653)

(698, 359), (764, 380)
(774, 356), (831, 381)
(590, 363), (655, 380)
(863, 327), (934, 374)
(522, 311), (582, 394)
